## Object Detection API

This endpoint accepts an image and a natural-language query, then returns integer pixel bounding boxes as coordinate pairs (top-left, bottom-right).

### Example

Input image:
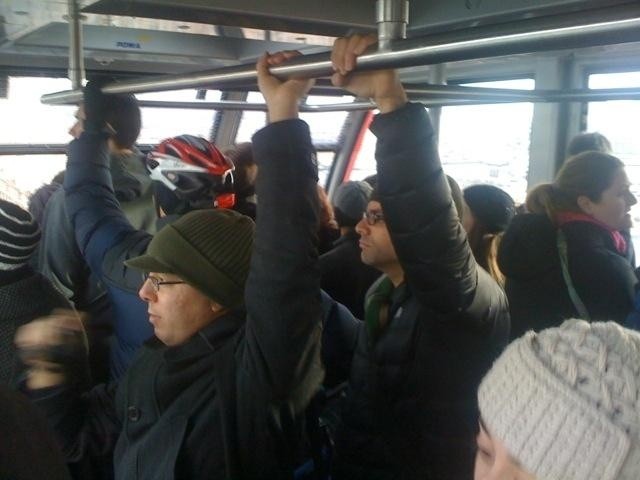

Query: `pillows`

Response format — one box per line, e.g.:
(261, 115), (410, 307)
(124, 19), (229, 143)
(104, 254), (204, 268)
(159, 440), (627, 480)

(147, 135), (236, 210)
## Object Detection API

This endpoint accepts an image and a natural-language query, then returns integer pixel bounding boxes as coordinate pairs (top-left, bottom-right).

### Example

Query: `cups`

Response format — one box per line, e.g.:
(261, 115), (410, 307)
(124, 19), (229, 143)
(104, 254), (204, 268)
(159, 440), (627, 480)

(141, 271), (186, 291)
(361, 212), (385, 223)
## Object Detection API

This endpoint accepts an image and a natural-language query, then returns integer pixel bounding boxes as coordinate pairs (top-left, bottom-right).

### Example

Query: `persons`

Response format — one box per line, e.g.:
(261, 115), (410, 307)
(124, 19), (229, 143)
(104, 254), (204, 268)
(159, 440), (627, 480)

(36, 81), (155, 308)
(318, 180), (383, 320)
(29, 185), (60, 222)
(294, 33), (511, 480)
(0, 199), (90, 480)
(66, 77), (234, 392)
(569, 133), (613, 157)
(473, 320), (640, 480)
(317, 183), (339, 256)
(13, 49), (320, 479)
(463, 185), (515, 257)
(496, 150), (640, 333)
(226, 142), (257, 220)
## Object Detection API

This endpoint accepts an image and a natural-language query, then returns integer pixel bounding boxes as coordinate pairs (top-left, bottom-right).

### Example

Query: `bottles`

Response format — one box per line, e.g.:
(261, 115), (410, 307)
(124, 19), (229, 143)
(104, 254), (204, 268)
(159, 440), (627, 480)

(334, 181), (374, 220)
(364, 173), (464, 224)
(123, 208), (256, 310)
(462, 185), (517, 234)
(0, 198), (41, 280)
(477, 317), (640, 480)
